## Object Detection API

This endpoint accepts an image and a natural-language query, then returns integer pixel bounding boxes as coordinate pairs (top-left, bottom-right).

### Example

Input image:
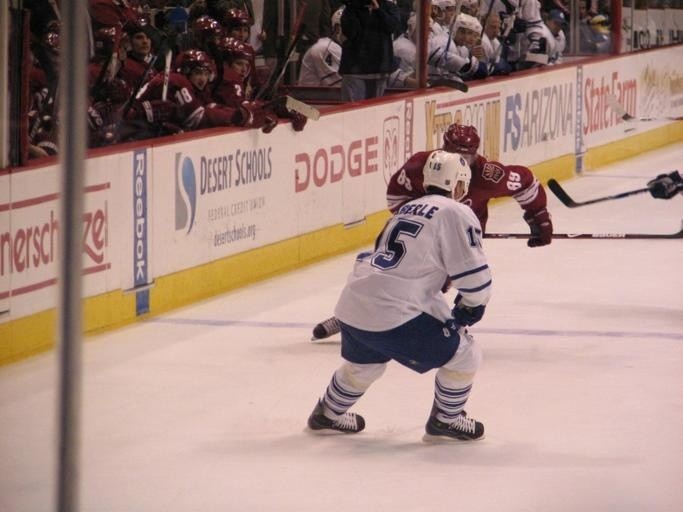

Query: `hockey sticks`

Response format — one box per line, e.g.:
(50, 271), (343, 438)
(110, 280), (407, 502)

(604, 95), (683, 123)
(261, 95), (321, 120)
(483, 219), (683, 239)
(143, 29), (173, 101)
(429, 79), (468, 91)
(547, 178), (652, 208)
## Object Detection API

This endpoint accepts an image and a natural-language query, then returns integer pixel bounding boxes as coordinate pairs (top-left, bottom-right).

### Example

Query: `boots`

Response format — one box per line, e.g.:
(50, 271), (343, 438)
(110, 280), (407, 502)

(313, 316), (342, 339)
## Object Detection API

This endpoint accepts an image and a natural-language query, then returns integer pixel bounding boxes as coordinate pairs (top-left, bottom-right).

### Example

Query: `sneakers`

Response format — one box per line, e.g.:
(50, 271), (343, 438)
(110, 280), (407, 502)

(308, 401), (365, 433)
(426, 410), (483, 439)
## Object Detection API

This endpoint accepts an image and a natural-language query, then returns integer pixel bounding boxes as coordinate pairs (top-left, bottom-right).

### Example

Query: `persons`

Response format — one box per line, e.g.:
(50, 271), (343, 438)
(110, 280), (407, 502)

(311, 120), (554, 342)
(645, 167), (683, 200)
(305, 149), (497, 444)
(27, 0), (614, 161)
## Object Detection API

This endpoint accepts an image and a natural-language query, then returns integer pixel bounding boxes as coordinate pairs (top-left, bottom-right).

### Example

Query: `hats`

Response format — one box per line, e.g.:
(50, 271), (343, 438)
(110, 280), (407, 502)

(548, 8), (567, 26)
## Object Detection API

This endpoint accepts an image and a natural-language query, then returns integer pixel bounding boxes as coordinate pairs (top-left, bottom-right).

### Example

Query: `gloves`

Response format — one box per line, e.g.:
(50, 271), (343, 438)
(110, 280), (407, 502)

(647, 170), (683, 199)
(453, 300), (485, 326)
(523, 210), (552, 247)
(235, 103), (306, 133)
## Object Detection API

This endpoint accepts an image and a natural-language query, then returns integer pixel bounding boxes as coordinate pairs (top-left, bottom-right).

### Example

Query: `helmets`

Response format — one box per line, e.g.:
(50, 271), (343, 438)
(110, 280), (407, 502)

(442, 124), (479, 153)
(333, 1), (483, 41)
(422, 151), (472, 193)
(45, 9), (255, 84)
